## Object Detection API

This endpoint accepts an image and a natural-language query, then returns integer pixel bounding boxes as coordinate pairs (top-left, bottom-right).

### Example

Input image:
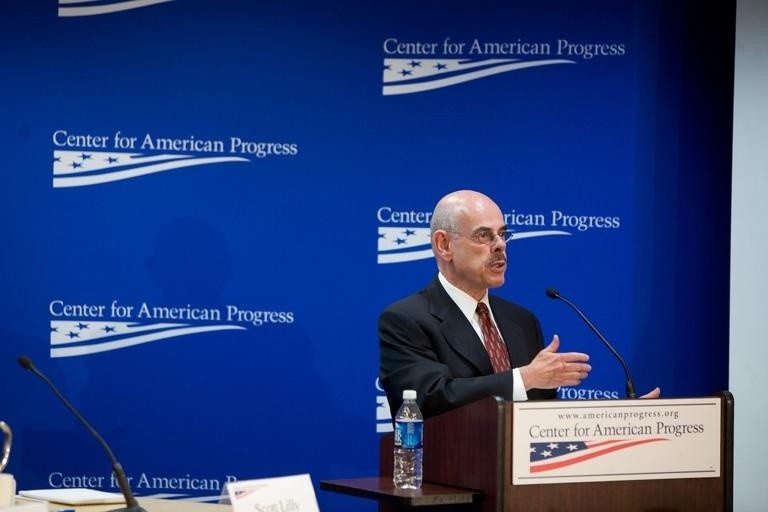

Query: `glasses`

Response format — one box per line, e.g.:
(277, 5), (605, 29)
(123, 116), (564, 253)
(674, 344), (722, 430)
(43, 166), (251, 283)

(450, 229), (513, 244)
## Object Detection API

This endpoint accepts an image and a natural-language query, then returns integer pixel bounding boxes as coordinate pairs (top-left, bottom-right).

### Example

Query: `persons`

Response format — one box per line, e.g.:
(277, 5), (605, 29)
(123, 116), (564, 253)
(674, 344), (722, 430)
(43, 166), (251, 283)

(379, 190), (660, 429)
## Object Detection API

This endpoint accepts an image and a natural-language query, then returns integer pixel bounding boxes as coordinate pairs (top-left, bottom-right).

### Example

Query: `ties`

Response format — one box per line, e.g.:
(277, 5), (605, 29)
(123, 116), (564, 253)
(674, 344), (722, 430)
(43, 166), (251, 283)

(475, 302), (513, 373)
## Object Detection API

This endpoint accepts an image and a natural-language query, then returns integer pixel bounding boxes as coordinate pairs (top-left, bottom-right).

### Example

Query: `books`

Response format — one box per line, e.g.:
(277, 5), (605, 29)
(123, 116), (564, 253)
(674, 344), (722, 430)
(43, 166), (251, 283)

(18, 489), (127, 512)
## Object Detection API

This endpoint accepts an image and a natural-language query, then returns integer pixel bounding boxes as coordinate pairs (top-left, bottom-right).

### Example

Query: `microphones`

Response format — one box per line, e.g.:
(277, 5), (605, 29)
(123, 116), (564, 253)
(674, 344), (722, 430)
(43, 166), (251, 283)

(18, 355), (146, 512)
(545, 288), (636, 399)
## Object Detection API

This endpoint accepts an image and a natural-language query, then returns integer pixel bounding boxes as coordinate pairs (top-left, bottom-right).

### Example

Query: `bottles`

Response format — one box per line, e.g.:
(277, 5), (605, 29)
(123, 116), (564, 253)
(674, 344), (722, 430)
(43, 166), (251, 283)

(393, 390), (426, 493)
